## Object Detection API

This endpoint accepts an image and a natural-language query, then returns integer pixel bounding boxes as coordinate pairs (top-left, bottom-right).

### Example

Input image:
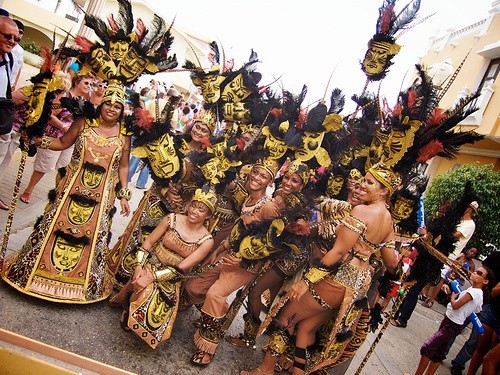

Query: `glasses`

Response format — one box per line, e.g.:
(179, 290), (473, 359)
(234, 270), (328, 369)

(80, 82), (93, 86)
(99, 85), (107, 89)
(474, 269), (486, 279)
(20, 31), (24, 34)
(0, 32), (18, 41)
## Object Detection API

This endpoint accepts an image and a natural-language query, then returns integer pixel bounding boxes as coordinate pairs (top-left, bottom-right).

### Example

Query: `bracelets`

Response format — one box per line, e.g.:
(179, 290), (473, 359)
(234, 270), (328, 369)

(116, 186), (132, 201)
(36, 136), (52, 150)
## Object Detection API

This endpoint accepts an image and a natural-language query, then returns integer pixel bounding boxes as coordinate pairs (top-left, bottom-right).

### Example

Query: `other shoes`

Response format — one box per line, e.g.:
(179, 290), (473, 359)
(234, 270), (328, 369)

(422, 299), (433, 308)
(0, 200), (9, 210)
(451, 365), (462, 375)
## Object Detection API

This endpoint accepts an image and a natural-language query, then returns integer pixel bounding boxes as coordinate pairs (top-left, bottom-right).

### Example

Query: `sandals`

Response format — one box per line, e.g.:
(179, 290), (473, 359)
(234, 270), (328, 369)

(190, 350), (215, 367)
(120, 307), (131, 332)
(390, 320), (407, 328)
(20, 191), (31, 204)
(239, 368), (267, 375)
(225, 335), (256, 350)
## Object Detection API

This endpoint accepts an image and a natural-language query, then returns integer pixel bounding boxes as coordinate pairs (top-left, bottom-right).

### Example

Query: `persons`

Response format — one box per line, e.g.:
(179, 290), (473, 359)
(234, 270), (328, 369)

(415, 265), (495, 375)
(232, 162), (401, 375)
(108, 187), (219, 349)
(0, 9), (500, 375)
(0, 83), (132, 305)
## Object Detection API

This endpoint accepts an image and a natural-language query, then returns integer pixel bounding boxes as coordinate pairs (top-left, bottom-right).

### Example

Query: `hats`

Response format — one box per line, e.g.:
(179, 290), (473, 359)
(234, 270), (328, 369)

(470, 201), (479, 215)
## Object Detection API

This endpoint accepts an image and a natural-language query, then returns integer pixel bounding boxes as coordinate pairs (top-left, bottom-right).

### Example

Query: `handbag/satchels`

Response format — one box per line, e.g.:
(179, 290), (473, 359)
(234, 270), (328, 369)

(0, 97), (15, 135)
